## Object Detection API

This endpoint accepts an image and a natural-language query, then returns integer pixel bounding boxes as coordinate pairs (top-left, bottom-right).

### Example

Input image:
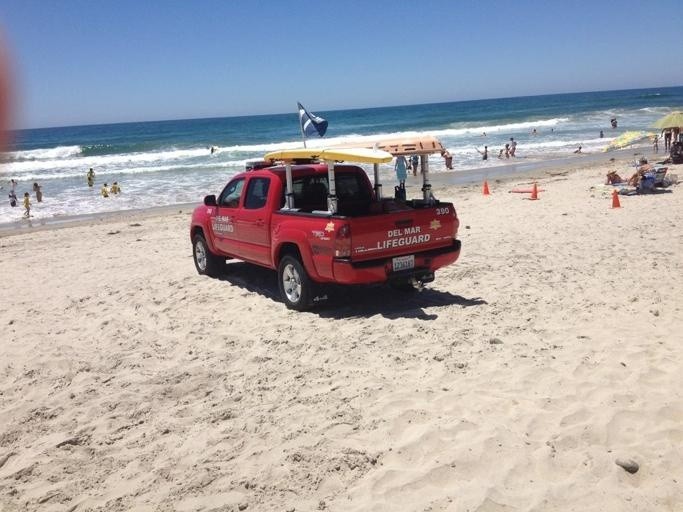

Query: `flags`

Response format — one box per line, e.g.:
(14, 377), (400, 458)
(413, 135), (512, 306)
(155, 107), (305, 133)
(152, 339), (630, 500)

(298, 103), (329, 142)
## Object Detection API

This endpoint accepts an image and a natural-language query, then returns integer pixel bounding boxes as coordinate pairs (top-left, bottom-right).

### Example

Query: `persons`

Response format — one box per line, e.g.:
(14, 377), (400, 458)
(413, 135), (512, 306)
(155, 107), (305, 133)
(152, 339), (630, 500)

(31, 182), (42, 202)
(10, 179), (17, 192)
(477, 137), (517, 160)
(411, 155), (419, 176)
(611, 118), (617, 129)
(110, 181), (120, 194)
(598, 130), (604, 138)
(86, 168), (96, 179)
(572, 146), (581, 153)
(531, 129), (538, 137)
(440, 151), (451, 170)
(101, 183), (109, 197)
(8, 190), (17, 206)
(87, 177), (94, 187)
(651, 127), (683, 154)
(23, 193), (31, 215)
(395, 156), (409, 187)
(605, 158), (617, 184)
(406, 159), (412, 173)
(625, 156), (654, 186)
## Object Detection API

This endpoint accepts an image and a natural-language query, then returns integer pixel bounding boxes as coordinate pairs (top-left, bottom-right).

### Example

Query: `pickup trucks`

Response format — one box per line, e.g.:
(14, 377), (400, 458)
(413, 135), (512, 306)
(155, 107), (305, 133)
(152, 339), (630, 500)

(188, 131), (462, 311)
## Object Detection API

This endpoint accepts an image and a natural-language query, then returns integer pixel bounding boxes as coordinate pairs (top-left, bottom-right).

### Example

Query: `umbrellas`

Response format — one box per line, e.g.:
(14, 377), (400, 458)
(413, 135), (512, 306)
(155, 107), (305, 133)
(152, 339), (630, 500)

(647, 111), (682, 130)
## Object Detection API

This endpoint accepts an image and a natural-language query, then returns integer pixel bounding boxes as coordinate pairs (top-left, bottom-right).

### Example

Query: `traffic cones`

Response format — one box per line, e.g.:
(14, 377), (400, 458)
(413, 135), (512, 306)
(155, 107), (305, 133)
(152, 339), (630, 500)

(610, 188), (620, 207)
(529, 182), (538, 200)
(482, 181), (489, 194)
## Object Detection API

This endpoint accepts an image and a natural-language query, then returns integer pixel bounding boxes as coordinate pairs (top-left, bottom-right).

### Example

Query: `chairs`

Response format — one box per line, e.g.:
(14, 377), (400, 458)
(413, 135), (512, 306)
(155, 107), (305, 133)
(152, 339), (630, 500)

(633, 166), (668, 191)
